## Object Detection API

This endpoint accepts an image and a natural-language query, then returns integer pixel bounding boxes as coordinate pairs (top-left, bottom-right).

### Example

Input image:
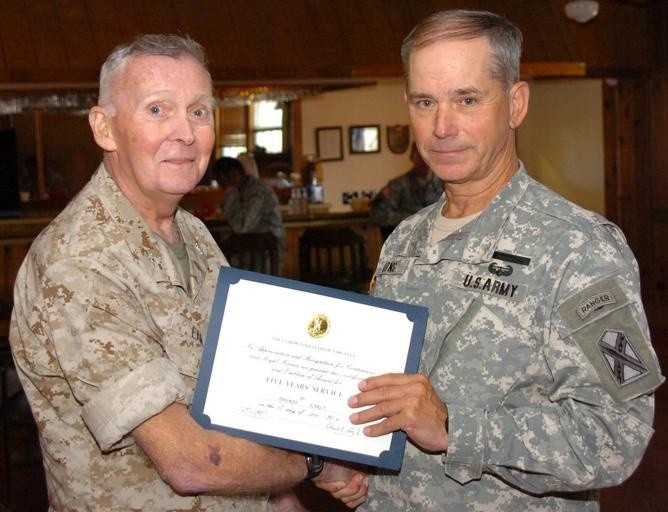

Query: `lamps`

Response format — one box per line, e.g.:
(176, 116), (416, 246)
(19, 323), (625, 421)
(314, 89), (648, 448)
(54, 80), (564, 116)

(564, 0), (600, 23)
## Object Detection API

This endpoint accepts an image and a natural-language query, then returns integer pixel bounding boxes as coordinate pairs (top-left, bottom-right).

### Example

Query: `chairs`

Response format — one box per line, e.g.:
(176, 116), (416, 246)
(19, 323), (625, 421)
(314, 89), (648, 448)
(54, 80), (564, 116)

(220, 232), (279, 276)
(298, 227), (373, 295)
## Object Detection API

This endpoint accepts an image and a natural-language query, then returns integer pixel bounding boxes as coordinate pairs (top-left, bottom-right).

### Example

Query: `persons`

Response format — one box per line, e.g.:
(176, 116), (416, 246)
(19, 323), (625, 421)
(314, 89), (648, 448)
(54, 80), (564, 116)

(372, 142), (444, 244)
(213, 156), (283, 257)
(314, 10), (666, 512)
(9, 31), (368, 511)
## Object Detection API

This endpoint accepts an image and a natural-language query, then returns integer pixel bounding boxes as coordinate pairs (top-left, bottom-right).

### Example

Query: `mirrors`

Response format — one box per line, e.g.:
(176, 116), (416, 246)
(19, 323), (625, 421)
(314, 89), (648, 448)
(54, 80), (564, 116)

(349, 125), (380, 153)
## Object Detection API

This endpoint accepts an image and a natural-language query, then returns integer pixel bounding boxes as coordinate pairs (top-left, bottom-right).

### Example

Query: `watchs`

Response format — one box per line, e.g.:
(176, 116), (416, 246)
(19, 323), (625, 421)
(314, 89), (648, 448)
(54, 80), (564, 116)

(304, 453), (324, 481)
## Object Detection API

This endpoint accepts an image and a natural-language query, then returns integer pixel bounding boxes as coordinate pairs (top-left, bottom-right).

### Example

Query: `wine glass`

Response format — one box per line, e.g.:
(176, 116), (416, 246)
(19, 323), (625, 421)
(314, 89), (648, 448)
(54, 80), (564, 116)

(0, 86), (318, 118)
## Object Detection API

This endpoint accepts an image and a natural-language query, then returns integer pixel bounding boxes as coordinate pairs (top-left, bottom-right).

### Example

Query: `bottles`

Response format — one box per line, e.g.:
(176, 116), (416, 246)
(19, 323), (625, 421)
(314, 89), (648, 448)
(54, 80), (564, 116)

(277, 171), (323, 218)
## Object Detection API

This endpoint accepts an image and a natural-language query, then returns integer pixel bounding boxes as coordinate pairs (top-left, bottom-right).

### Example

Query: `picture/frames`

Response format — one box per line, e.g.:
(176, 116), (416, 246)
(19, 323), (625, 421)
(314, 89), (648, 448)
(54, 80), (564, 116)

(316, 127), (343, 161)
